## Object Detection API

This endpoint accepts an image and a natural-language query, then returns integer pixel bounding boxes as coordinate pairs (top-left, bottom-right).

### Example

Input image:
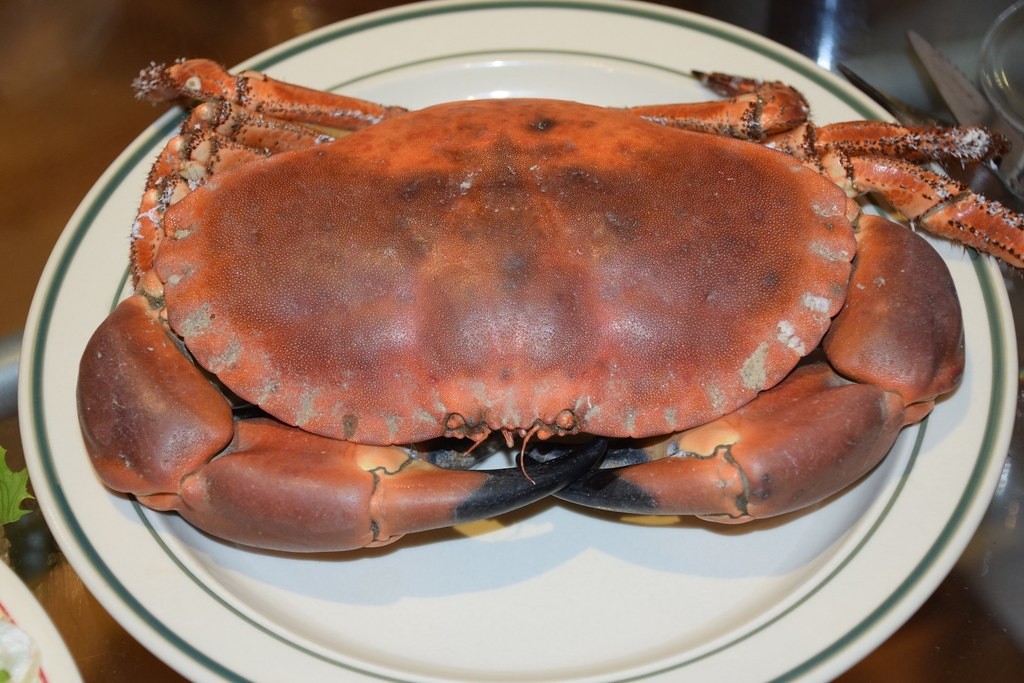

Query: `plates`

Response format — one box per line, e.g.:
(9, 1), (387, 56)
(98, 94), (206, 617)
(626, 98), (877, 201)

(18, 0), (1018, 683)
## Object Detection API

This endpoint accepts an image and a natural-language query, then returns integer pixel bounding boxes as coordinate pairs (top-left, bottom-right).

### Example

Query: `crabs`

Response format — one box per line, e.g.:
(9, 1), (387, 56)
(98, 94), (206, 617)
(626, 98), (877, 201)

(71, 58), (1024, 556)
